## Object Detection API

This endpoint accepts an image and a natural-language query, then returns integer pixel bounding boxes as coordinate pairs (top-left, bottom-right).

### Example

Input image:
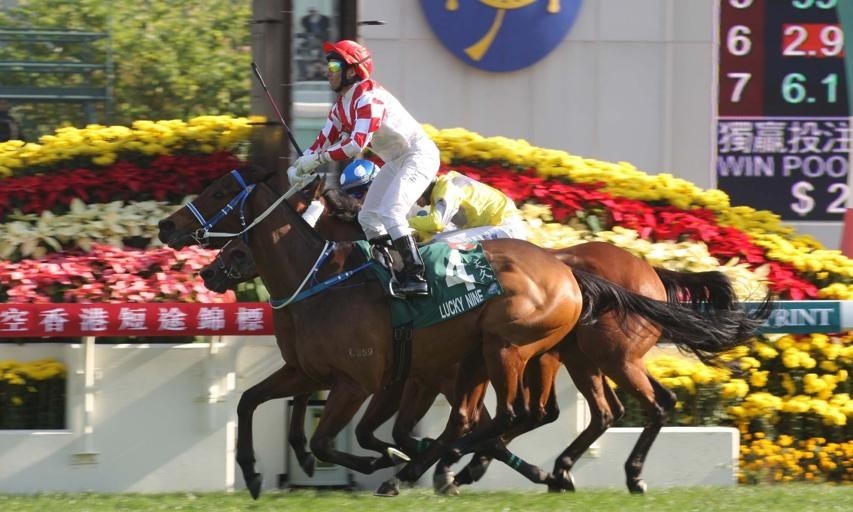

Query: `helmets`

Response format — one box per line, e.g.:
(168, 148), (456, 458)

(323, 40), (373, 79)
(340, 159), (381, 190)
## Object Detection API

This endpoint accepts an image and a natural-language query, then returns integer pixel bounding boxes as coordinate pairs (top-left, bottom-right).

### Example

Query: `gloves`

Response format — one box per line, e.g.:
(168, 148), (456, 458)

(299, 149), (321, 172)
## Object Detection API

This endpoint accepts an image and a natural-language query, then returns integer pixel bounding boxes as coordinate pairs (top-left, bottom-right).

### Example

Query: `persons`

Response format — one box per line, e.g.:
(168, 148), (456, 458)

(337, 156), (459, 242)
(403, 168), (527, 249)
(284, 38), (442, 297)
(294, 6), (333, 80)
(0, 99), (23, 146)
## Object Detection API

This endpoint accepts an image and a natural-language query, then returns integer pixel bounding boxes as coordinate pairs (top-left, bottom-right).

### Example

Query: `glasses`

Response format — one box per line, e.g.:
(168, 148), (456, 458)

(351, 191), (365, 199)
(328, 62), (346, 73)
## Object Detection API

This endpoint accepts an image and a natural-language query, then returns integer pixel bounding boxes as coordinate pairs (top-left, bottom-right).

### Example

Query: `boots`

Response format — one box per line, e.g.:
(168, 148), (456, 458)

(369, 234), (428, 297)
